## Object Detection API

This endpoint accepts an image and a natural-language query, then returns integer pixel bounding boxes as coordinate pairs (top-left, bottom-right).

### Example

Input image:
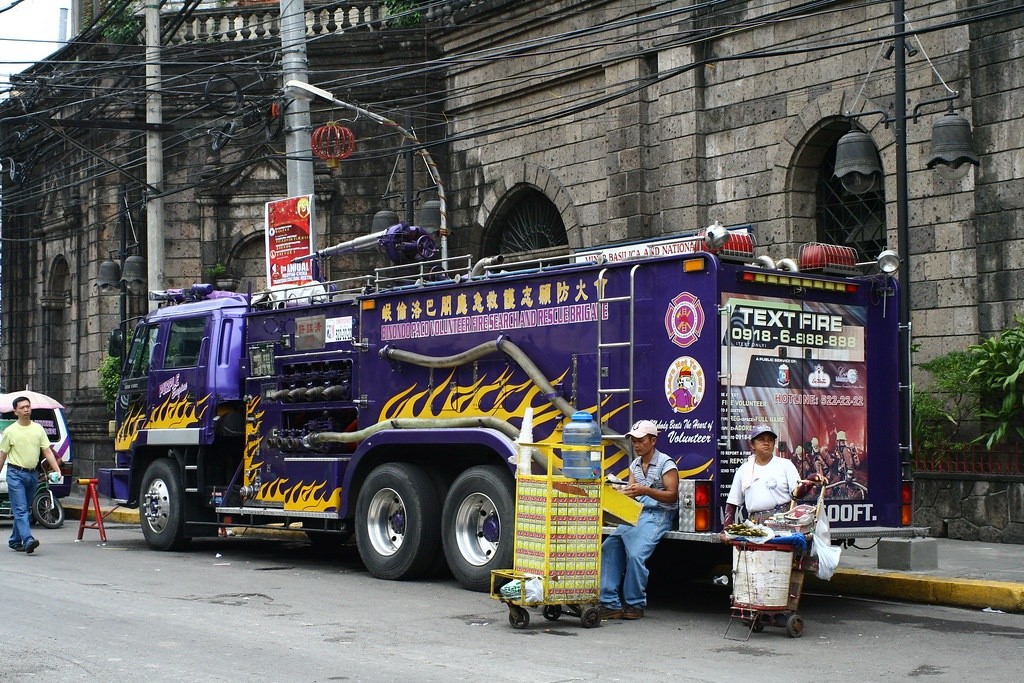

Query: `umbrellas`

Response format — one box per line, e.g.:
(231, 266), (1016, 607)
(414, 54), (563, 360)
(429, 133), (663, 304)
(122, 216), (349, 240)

(0, 383), (65, 413)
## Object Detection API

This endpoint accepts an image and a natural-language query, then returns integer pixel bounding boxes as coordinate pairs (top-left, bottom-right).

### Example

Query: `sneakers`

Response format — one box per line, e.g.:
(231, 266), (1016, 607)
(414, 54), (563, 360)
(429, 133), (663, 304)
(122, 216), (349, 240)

(25, 538), (39, 553)
(623, 605), (644, 619)
(597, 606), (622, 619)
(9, 543), (25, 551)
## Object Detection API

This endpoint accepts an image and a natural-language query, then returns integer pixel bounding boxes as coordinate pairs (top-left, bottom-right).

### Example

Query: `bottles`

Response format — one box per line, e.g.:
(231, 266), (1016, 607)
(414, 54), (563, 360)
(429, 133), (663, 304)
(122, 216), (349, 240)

(562, 411), (601, 480)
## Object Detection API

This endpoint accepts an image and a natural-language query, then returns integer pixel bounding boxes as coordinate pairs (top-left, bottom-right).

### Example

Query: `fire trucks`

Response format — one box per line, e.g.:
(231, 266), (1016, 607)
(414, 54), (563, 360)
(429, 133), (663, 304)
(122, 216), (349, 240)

(97, 224), (916, 592)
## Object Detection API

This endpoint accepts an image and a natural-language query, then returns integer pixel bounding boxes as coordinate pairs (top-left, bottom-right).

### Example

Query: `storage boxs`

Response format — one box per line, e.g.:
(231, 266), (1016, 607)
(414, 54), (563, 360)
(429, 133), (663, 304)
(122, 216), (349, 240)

(98, 450), (130, 499)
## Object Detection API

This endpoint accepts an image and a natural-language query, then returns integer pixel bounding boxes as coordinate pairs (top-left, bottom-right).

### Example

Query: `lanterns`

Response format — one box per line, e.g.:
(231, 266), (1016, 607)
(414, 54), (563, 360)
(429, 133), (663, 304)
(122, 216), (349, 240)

(311, 119), (356, 168)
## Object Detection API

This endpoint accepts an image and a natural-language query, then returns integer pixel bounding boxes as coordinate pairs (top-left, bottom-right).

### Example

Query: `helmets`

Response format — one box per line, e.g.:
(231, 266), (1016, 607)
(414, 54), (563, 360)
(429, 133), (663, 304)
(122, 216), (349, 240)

(796, 445), (806, 460)
(836, 431), (849, 441)
(811, 438), (820, 453)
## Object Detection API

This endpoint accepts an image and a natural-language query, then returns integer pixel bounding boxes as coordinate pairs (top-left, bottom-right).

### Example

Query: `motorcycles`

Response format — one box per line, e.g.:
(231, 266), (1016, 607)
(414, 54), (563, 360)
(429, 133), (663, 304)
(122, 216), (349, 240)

(0, 390), (74, 529)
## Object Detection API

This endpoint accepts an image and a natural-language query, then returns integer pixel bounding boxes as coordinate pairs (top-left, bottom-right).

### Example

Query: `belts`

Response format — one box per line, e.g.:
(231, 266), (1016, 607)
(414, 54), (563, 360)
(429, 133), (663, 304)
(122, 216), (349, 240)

(7, 462), (35, 473)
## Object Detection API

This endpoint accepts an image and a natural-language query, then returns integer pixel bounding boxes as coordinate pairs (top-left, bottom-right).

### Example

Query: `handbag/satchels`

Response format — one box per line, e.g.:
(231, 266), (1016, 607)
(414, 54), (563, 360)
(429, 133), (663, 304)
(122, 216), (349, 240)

(809, 532), (842, 580)
(810, 498), (832, 556)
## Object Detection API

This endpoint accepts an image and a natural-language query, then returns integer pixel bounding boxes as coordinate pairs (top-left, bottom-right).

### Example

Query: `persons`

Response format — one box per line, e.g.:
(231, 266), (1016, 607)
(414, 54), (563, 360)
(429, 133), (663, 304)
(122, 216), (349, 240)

(0, 397), (61, 554)
(721, 425), (829, 534)
(598, 419), (679, 620)
(790, 430), (864, 499)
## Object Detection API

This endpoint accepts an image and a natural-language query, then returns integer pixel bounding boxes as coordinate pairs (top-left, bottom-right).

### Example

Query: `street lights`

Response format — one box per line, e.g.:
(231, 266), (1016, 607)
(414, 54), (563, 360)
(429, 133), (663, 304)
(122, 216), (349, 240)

(93, 242), (148, 368)
(285, 79), (450, 271)
(371, 183), (445, 234)
(833, 90), (984, 527)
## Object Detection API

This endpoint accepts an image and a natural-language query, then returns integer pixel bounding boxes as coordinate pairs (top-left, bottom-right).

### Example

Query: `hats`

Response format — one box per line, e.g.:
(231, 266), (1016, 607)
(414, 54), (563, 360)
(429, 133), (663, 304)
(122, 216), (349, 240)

(624, 420), (658, 438)
(751, 424), (777, 441)
